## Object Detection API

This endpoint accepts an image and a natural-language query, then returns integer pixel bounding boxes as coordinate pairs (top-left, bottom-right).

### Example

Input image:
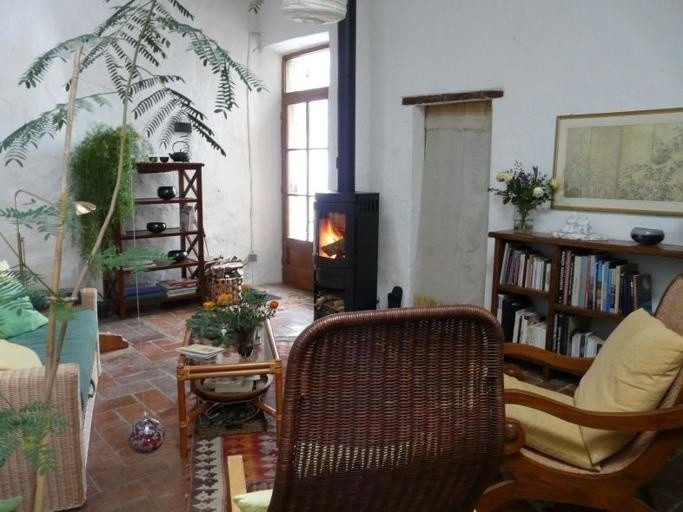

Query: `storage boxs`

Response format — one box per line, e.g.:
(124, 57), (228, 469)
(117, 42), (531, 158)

(205, 270), (245, 308)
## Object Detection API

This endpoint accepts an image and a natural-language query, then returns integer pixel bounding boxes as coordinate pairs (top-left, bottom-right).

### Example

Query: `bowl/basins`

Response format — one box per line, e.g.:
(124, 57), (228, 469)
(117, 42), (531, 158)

(158, 186), (177, 200)
(147, 223), (166, 233)
(631, 227), (664, 246)
(168, 250), (187, 263)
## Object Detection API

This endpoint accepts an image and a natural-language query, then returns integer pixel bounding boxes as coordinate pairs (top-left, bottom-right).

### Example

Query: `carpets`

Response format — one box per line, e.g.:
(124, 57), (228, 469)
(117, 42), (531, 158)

(183, 334), (301, 512)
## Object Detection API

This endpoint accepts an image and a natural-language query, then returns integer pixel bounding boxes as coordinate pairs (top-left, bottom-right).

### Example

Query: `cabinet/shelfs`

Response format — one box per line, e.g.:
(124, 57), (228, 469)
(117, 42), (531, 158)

(66, 121), (155, 283)
(102, 162), (207, 321)
(487, 227), (683, 383)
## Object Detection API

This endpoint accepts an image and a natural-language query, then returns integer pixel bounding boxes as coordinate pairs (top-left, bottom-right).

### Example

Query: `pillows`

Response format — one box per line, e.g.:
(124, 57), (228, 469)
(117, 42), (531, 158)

(573, 302), (682, 464)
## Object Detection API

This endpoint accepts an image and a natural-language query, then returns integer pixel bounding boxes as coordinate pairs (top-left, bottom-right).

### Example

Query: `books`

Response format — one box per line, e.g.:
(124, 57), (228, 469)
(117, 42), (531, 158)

(156, 277), (199, 300)
(171, 343), (225, 361)
(556, 249), (654, 316)
(202, 374), (261, 396)
(553, 312), (606, 359)
(125, 282), (167, 305)
(497, 241), (551, 293)
(496, 294), (546, 350)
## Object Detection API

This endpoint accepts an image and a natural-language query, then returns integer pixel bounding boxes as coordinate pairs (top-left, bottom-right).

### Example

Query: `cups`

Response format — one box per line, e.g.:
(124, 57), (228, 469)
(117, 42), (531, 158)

(160, 157), (169, 162)
(148, 157), (157, 162)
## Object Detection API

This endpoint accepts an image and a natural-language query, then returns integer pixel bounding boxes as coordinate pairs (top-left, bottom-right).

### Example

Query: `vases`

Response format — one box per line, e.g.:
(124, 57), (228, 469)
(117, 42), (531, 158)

(512, 204), (535, 234)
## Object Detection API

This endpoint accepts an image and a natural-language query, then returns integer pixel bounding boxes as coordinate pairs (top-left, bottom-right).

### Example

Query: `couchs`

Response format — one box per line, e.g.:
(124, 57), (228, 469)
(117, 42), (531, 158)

(0, 287), (103, 512)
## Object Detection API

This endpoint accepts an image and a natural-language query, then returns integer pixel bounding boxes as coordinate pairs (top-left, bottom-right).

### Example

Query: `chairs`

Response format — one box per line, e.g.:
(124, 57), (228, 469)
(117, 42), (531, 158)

(474, 272), (683, 512)
(225, 303), (507, 512)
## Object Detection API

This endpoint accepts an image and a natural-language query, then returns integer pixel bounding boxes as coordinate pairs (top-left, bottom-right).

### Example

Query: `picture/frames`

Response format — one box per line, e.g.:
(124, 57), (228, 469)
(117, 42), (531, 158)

(548, 105), (683, 218)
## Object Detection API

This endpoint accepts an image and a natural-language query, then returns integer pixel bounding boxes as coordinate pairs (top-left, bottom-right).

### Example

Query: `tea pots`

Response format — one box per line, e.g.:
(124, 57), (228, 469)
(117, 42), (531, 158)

(168, 140), (190, 163)
(130, 416), (165, 453)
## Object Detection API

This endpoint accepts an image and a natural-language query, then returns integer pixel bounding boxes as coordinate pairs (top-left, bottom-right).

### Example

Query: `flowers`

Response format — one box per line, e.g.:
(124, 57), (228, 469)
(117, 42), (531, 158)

(487, 158), (564, 229)
